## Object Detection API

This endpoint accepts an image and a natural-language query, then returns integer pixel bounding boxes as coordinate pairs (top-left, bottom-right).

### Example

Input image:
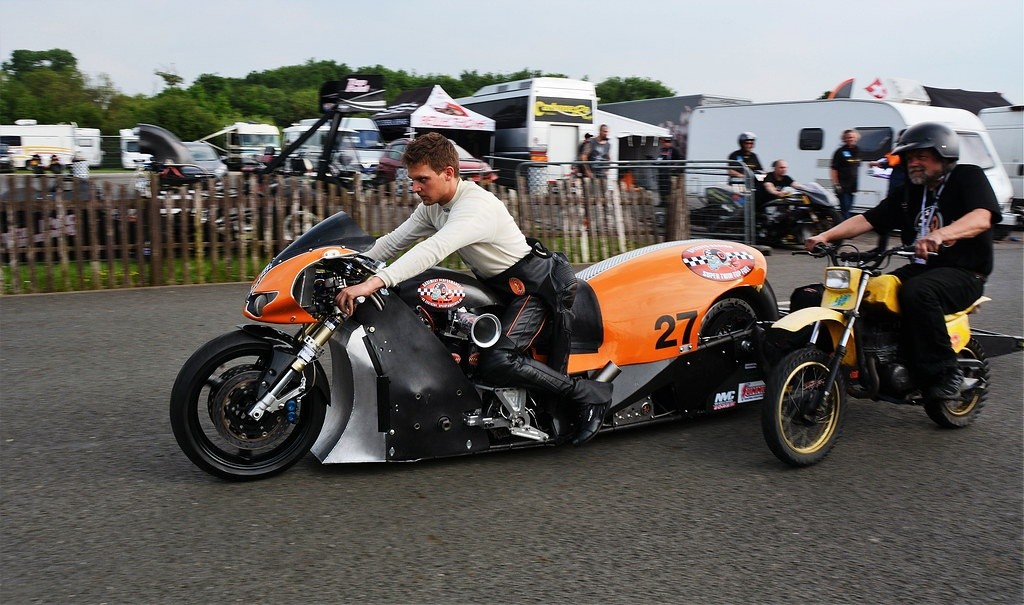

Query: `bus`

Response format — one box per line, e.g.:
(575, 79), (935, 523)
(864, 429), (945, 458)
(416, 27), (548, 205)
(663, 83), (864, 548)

(100, 77), (499, 263)
(450, 76), (602, 196)
(598, 95), (755, 192)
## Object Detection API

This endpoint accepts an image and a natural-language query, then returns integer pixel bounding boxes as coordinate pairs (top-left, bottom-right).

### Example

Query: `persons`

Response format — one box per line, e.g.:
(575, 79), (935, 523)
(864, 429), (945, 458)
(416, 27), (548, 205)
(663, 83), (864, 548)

(866, 128), (916, 251)
(581, 124), (611, 227)
(578, 133), (593, 173)
(805, 122), (1004, 400)
(727, 132), (764, 184)
(829, 130), (865, 222)
(708, 159), (818, 233)
(646, 137), (677, 207)
(334, 132), (613, 447)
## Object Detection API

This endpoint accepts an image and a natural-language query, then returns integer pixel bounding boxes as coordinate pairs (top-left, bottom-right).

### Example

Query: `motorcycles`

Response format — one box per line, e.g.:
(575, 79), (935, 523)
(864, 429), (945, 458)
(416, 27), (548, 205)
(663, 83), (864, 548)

(760, 241), (994, 468)
(168, 208), (783, 483)
(689, 181), (845, 257)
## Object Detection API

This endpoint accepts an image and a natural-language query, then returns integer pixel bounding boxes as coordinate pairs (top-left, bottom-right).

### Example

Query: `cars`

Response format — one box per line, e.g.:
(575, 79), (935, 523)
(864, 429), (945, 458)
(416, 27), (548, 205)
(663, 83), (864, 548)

(380, 136), (496, 188)
(0, 171), (108, 263)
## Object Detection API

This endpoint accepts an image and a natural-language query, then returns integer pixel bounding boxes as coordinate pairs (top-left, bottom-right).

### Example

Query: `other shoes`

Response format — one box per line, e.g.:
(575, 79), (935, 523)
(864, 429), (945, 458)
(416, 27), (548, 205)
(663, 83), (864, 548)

(936, 365), (964, 400)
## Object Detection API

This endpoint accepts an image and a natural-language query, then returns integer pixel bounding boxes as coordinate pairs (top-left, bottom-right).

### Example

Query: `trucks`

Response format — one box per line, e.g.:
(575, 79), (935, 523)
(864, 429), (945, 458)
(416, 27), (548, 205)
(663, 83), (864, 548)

(977, 106), (1024, 232)
(684, 100), (1020, 245)
(0, 119), (105, 170)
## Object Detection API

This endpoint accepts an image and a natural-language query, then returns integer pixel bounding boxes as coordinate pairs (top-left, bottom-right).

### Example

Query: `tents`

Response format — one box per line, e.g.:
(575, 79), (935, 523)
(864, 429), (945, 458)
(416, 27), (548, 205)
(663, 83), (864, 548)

(370, 85), (496, 170)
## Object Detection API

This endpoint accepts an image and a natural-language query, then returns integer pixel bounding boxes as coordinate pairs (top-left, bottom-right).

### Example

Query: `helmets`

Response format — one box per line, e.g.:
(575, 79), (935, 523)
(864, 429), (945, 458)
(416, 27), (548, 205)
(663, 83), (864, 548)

(891, 123), (959, 160)
(738, 134), (756, 147)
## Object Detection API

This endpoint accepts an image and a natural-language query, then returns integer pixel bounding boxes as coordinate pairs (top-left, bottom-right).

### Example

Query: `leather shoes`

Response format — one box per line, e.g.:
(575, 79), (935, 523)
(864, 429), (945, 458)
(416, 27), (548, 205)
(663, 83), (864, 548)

(571, 398), (612, 446)
